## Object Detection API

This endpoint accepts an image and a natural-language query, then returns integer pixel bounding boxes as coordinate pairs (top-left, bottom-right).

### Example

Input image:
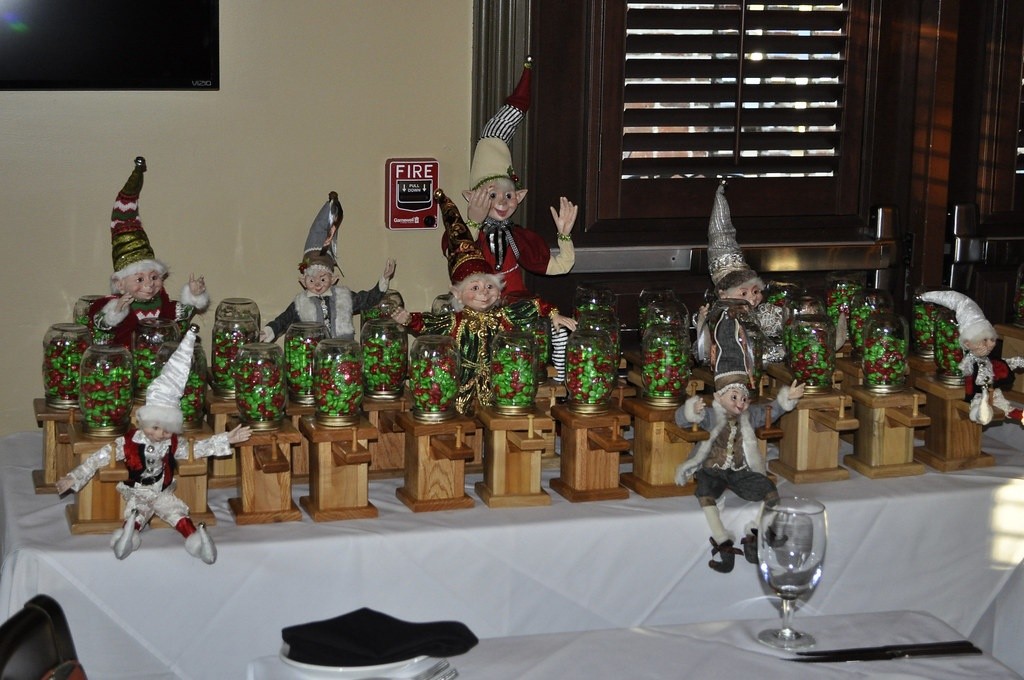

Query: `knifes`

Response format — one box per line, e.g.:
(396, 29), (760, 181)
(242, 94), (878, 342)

(782, 639), (980, 664)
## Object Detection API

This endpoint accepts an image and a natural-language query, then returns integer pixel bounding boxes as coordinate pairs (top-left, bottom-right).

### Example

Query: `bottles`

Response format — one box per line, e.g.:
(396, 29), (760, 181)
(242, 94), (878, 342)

(40, 283), (975, 433)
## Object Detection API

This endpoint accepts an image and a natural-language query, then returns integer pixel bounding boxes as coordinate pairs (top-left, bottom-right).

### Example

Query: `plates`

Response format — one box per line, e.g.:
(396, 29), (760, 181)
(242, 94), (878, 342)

(278, 639), (430, 679)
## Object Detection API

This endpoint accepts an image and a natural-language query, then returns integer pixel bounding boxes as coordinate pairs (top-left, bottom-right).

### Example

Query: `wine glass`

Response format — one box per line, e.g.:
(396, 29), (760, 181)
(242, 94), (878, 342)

(754, 496), (828, 652)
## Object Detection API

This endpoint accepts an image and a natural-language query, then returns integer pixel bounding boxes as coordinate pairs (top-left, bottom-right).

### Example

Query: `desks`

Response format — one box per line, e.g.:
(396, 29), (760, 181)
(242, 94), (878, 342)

(243, 608), (1022, 678)
(1, 429), (1022, 679)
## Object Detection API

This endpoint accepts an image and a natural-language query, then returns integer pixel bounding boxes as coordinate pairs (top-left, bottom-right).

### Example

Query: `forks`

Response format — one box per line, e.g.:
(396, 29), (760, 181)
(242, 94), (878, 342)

(409, 657), (458, 680)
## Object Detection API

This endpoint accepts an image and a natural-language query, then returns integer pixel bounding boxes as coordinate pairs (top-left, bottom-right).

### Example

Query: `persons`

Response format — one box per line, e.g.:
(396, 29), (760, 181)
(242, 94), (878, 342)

(691, 271), (788, 368)
(56, 405), (253, 564)
(257, 252), (395, 343)
(957, 324), (1024, 425)
(676, 380), (806, 573)
(91, 254), (209, 349)
(441, 170), (579, 404)
(390, 270), (578, 414)
